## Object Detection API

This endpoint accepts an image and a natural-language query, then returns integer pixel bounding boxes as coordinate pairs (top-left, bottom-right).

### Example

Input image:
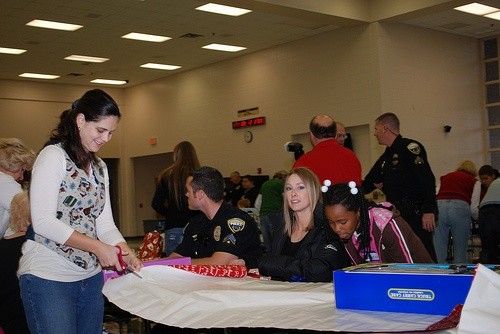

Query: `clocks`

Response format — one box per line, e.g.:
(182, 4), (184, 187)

(244, 131), (253, 143)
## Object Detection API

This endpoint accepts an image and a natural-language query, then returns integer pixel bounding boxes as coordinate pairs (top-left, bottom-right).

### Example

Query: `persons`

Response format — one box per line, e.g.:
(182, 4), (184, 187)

(0, 138), (37, 334)
(18, 89), (144, 334)
(332, 122), (347, 146)
(260, 168), (338, 282)
(364, 114), (439, 264)
(161, 167), (261, 273)
(153, 141), (203, 256)
(437, 160), (500, 262)
(291, 115), (362, 186)
(323, 177), (435, 264)
(224, 171), (289, 248)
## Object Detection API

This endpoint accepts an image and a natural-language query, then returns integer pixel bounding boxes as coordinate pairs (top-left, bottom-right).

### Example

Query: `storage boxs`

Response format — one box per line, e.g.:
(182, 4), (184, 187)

(333, 260), (500, 316)
(103, 257), (192, 284)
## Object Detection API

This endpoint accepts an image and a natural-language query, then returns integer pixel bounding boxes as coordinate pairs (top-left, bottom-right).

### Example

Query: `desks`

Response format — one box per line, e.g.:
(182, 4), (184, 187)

(104, 278), (458, 334)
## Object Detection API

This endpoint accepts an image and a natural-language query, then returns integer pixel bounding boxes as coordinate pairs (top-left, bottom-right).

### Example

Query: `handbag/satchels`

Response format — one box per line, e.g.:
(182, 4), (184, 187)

(140, 231), (162, 259)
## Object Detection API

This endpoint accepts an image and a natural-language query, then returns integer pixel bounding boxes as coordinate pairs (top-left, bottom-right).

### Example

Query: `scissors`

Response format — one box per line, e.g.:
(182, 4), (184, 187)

(110, 245), (144, 280)
